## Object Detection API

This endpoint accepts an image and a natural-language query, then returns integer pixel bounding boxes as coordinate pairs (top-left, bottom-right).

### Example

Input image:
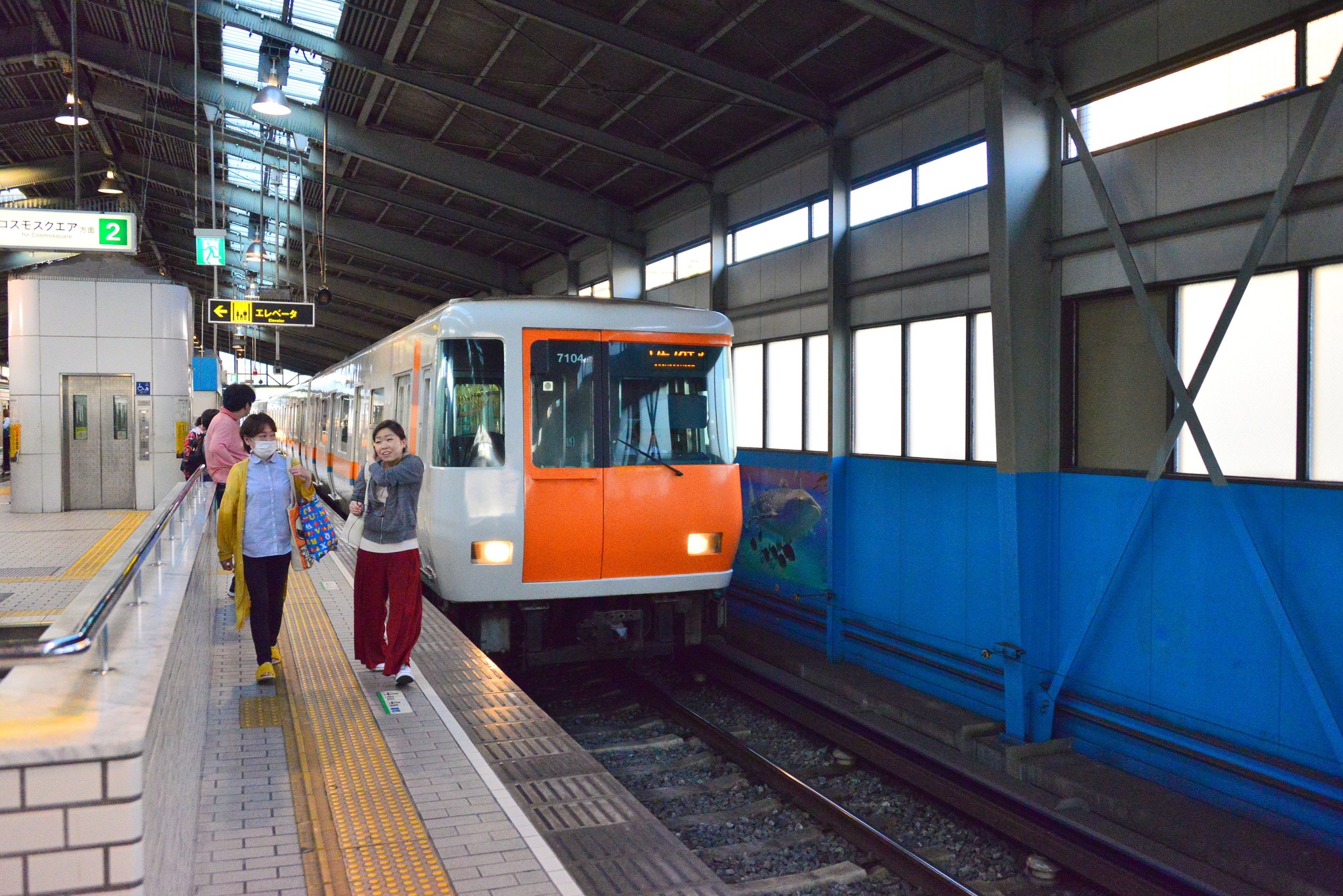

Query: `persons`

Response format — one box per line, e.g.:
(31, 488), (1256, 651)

(204, 384), (258, 600)
(182, 415), (205, 482)
(215, 411), (316, 684)
(620, 420), (668, 454)
(2, 409), (11, 474)
(197, 409), (220, 482)
(349, 419), (424, 686)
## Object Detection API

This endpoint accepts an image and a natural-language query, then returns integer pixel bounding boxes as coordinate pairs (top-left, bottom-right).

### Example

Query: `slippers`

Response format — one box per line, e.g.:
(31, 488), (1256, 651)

(270, 646), (281, 665)
(256, 662), (276, 682)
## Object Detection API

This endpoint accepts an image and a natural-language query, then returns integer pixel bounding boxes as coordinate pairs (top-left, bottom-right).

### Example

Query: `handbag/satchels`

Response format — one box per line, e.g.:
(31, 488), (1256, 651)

(286, 455), (315, 572)
(3, 426), (10, 438)
(299, 489), (338, 563)
(339, 462), (372, 551)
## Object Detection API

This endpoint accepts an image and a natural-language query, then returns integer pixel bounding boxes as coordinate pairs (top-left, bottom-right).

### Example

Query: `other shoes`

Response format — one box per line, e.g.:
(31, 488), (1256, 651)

(3, 470), (10, 474)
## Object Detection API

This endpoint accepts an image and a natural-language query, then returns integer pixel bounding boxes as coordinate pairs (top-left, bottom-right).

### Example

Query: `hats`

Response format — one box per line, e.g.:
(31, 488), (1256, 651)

(203, 409), (221, 429)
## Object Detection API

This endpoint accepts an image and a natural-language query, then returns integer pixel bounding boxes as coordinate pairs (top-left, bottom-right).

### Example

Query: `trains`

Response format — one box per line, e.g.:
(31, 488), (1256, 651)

(266, 297), (744, 678)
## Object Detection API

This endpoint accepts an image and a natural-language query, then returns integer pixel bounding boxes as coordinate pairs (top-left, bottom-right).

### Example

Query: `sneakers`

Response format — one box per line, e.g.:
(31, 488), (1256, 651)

(227, 577), (235, 597)
(371, 662), (385, 671)
(395, 663), (414, 685)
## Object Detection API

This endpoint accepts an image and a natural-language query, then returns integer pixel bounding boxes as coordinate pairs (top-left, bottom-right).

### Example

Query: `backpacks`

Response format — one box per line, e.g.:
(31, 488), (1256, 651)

(188, 433), (207, 470)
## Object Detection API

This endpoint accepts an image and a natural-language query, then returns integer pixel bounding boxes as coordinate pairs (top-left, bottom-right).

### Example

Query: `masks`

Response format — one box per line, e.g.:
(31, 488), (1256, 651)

(248, 437), (278, 459)
